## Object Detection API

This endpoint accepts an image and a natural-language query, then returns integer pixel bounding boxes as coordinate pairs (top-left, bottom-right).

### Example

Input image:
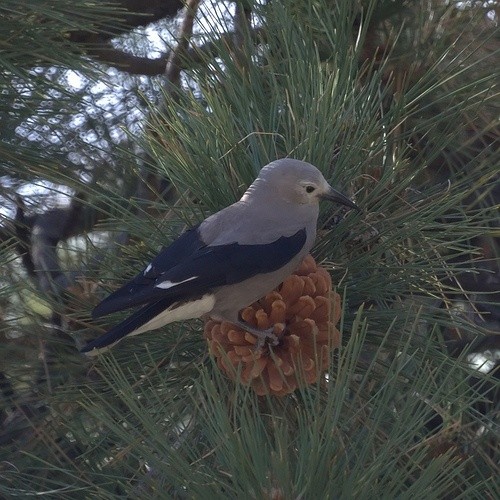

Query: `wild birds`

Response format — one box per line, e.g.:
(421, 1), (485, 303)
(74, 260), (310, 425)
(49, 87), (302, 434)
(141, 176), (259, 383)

(79, 158), (364, 356)
(0, 192), (96, 291)
